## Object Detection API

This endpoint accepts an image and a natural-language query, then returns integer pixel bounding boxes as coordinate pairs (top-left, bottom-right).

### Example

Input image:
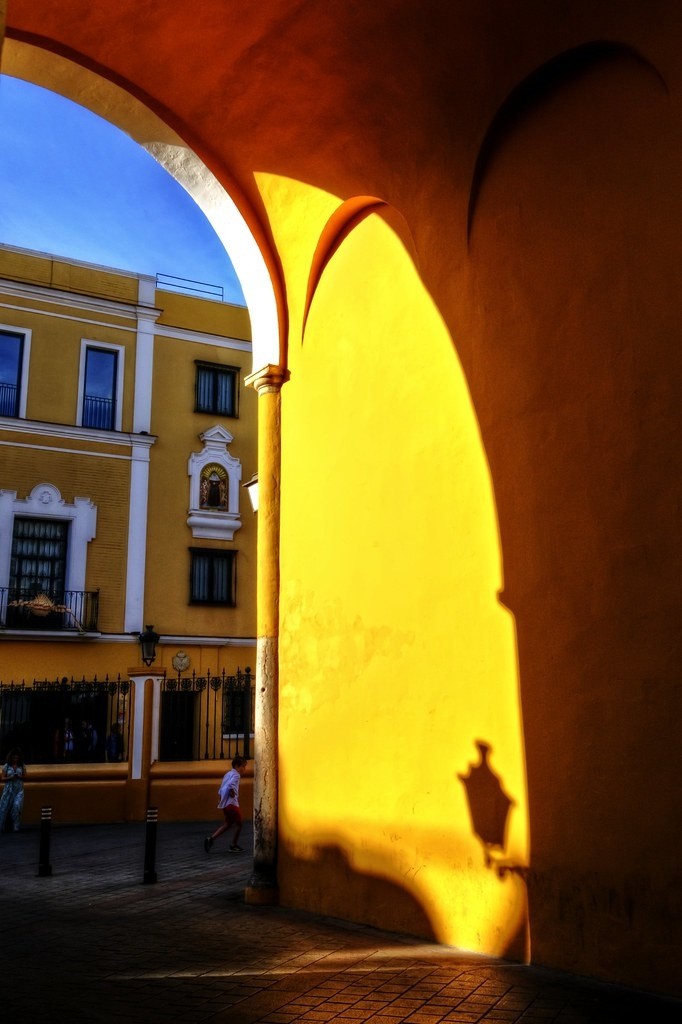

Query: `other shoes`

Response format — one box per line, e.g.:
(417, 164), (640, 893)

(13, 824), (20, 832)
(228, 844), (248, 853)
(205, 836), (213, 853)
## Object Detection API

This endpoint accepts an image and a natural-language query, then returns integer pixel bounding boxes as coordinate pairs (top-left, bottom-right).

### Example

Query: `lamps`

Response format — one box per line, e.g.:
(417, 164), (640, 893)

(241, 471), (259, 514)
(140, 624), (160, 667)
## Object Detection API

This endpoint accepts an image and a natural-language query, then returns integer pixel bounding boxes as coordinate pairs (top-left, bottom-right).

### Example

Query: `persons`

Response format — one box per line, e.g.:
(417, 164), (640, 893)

(0, 749), (27, 832)
(79, 718), (98, 762)
(55, 716), (74, 763)
(106, 723), (124, 761)
(205, 756), (247, 852)
